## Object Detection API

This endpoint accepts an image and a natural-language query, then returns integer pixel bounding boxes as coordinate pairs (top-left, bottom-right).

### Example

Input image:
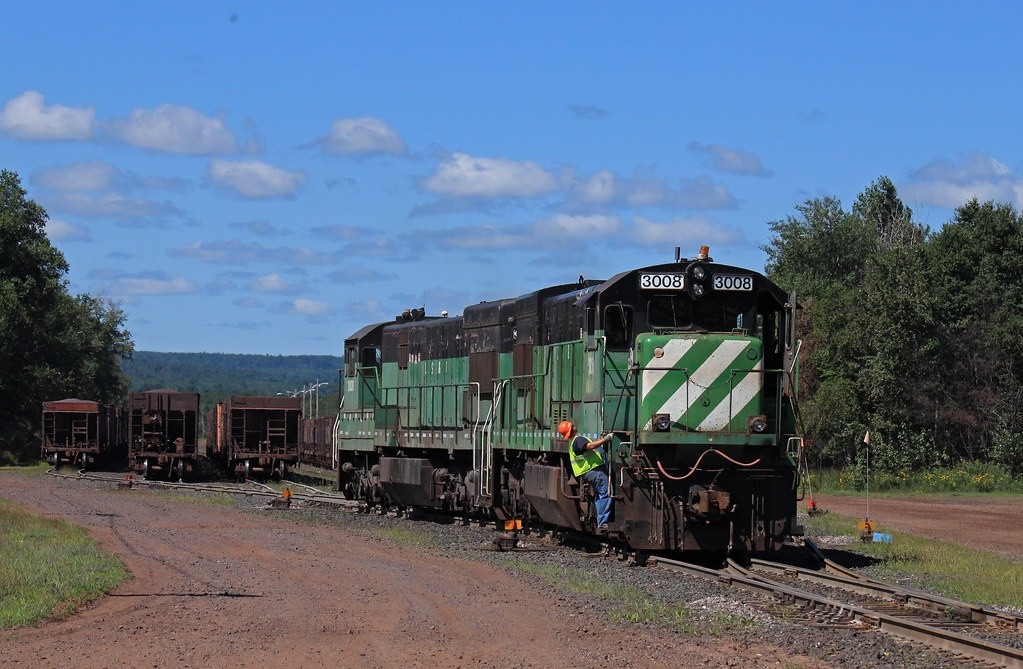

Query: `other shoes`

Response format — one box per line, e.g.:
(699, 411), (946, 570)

(598, 524), (609, 530)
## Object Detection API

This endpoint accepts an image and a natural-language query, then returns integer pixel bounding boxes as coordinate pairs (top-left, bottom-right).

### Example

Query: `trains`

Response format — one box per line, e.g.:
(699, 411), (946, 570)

(331, 245), (806, 556)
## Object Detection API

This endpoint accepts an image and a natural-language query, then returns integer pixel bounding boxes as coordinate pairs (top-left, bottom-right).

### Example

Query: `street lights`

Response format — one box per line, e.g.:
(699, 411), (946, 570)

(312, 378), (329, 418)
(277, 385), (305, 419)
(305, 382), (315, 419)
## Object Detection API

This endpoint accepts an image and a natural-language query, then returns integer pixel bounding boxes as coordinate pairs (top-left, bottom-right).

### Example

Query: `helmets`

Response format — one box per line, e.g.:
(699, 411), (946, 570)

(558, 421), (573, 440)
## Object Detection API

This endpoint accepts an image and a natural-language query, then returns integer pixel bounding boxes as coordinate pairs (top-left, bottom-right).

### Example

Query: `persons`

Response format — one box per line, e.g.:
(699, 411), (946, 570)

(558, 421), (614, 530)
